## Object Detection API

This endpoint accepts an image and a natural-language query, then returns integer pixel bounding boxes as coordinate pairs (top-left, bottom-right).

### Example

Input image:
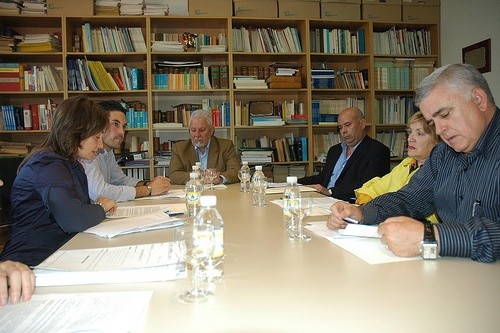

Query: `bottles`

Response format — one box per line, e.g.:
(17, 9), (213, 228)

(282, 176), (302, 232)
(251, 166), (266, 206)
(241, 160), (250, 192)
(186, 173), (203, 218)
(191, 166), (201, 184)
(195, 162), (204, 183)
(194, 195), (225, 295)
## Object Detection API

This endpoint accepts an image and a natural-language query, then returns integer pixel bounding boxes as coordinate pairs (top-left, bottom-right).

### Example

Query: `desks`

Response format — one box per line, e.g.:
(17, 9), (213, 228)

(0, 183), (500, 333)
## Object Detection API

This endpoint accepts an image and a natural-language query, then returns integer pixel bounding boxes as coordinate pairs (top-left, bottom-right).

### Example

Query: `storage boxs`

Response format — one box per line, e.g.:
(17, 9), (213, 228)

(189, 0), (441, 25)
(47, 0), (95, 17)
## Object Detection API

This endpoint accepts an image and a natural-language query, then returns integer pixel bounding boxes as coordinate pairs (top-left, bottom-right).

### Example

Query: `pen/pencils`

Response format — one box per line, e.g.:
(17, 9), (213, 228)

(321, 208), (360, 223)
(472, 198), (480, 218)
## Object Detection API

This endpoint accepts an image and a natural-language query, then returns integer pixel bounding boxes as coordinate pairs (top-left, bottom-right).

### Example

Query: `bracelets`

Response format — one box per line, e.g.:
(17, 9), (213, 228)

(93, 202), (105, 212)
(144, 181), (150, 185)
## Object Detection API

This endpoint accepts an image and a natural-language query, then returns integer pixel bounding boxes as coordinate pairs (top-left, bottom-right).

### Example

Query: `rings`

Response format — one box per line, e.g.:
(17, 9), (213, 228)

(386, 244), (389, 249)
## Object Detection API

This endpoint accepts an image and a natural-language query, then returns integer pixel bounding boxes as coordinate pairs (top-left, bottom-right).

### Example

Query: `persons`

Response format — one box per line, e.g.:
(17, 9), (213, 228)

(169, 110), (240, 184)
(298, 107), (391, 204)
(327, 64), (500, 262)
(79, 100), (170, 201)
(0, 97), (117, 269)
(354, 111), (439, 224)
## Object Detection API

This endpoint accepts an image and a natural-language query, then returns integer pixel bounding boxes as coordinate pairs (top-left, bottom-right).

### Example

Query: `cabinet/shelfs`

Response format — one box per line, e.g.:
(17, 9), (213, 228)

(0, 17), (441, 188)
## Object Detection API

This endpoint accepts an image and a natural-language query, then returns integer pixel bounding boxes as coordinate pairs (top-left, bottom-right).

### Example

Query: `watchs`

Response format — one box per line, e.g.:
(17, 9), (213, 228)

(146, 185), (152, 196)
(421, 221), (438, 260)
(328, 189), (332, 194)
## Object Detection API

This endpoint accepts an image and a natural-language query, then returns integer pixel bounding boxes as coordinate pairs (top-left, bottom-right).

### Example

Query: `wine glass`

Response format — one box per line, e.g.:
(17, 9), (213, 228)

(252, 177), (267, 207)
(288, 192), (313, 241)
(173, 223), (215, 303)
(205, 168), (217, 191)
(238, 170), (250, 192)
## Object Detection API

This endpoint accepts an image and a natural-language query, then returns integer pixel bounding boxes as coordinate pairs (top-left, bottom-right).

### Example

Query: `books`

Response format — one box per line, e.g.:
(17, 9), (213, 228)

(0, 32), (63, 157)
(94, 0), (169, 15)
(311, 28), (369, 173)
(68, 23), (148, 181)
(233, 27), (308, 182)
(0, 0), (47, 15)
(373, 27), (437, 157)
(152, 33), (231, 178)
